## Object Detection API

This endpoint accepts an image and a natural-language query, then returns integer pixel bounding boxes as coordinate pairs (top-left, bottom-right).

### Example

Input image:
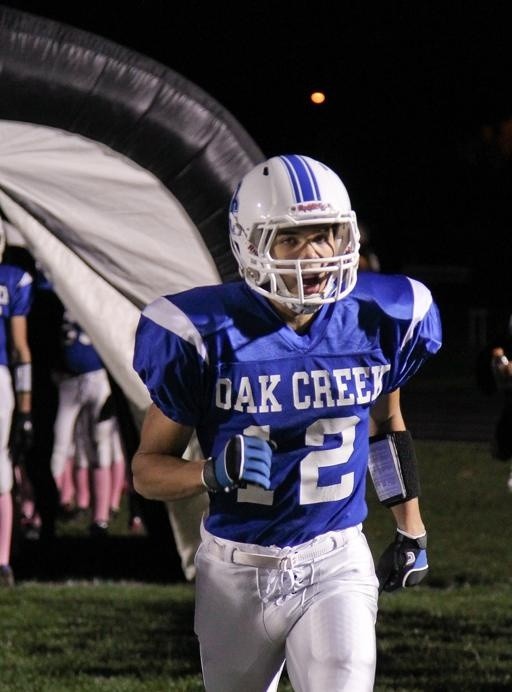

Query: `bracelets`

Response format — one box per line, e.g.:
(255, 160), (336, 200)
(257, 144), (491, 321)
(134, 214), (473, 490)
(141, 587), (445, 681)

(366, 430), (421, 508)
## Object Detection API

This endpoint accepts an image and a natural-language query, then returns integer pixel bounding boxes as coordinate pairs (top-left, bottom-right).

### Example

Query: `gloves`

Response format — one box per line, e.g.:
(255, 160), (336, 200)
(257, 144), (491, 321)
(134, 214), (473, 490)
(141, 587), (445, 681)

(202, 431), (278, 493)
(375, 529), (432, 592)
(11, 410), (34, 450)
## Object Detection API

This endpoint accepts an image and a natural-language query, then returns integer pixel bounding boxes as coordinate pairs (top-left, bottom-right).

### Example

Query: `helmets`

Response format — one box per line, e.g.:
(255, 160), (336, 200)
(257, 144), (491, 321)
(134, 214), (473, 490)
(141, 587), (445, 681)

(229, 155), (361, 313)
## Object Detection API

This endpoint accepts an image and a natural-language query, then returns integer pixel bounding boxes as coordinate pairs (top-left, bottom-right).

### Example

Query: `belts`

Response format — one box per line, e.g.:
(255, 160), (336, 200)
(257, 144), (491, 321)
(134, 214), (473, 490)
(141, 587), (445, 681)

(205, 527), (356, 569)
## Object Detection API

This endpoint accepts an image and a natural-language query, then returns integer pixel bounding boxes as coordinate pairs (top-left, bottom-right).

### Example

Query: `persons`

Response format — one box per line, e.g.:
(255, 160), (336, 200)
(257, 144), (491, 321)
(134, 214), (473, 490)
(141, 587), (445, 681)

(486, 289), (512, 461)
(131, 153), (442, 692)
(2, 215), (143, 588)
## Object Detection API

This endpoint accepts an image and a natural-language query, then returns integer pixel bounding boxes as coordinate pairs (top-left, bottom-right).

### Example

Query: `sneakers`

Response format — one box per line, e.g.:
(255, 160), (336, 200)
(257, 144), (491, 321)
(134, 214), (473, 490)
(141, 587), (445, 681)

(0, 498), (119, 589)
